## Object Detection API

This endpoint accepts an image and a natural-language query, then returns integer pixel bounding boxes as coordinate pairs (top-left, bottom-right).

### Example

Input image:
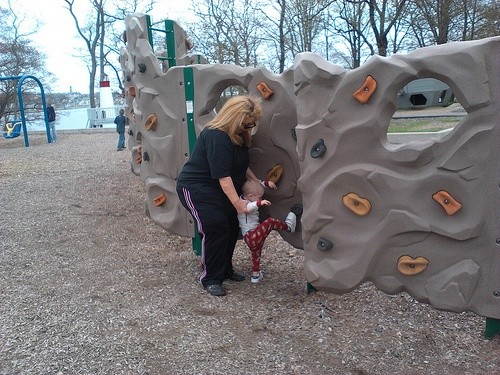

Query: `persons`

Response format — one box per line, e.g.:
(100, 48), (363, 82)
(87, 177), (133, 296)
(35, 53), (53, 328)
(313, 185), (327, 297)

(114, 109), (127, 151)
(176, 96), (262, 296)
(46, 103), (58, 143)
(237, 179), (296, 283)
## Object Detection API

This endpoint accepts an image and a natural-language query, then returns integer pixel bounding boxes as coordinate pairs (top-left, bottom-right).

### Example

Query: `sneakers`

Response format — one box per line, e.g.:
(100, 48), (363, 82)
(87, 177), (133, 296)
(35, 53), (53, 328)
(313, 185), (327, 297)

(285, 211), (297, 234)
(230, 271), (244, 281)
(206, 284), (227, 297)
(251, 271), (263, 284)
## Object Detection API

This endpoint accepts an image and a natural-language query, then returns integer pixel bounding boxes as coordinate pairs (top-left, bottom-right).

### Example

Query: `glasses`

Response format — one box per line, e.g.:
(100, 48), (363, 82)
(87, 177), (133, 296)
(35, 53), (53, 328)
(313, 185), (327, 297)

(242, 122), (256, 129)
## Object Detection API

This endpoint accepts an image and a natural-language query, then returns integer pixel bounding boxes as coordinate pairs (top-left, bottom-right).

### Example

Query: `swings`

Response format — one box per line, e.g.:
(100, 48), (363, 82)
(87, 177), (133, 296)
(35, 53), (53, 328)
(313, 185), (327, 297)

(3, 79), (21, 139)
